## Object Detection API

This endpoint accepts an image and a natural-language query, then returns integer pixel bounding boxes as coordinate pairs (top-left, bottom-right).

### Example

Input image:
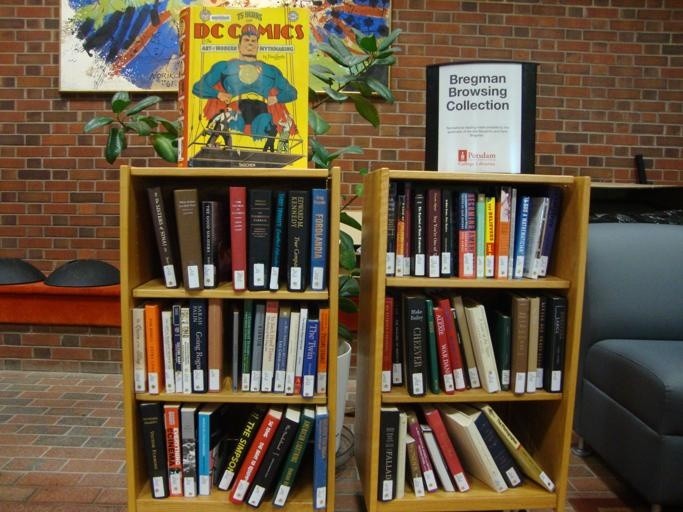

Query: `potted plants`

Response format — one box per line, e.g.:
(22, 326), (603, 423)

(86, 29), (402, 470)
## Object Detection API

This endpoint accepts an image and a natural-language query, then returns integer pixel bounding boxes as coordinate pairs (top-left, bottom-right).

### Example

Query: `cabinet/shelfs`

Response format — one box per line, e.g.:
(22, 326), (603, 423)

(352, 165), (589, 511)
(114, 165), (342, 512)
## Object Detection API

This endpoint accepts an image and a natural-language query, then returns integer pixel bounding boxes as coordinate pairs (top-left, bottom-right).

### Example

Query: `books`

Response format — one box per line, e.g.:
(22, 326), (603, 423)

(424, 62), (539, 175)
(378, 400), (556, 502)
(176, 4), (308, 171)
(385, 179), (560, 279)
(133, 298), (329, 397)
(148, 186), (329, 292)
(139, 401), (329, 508)
(381, 293), (567, 395)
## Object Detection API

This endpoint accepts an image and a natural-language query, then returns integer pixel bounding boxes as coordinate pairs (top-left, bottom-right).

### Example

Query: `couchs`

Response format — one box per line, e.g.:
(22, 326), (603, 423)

(578, 220), (683, 507)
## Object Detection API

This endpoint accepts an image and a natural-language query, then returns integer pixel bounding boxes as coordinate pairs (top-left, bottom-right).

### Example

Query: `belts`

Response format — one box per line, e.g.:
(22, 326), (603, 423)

(228, 92), (268, 105)
